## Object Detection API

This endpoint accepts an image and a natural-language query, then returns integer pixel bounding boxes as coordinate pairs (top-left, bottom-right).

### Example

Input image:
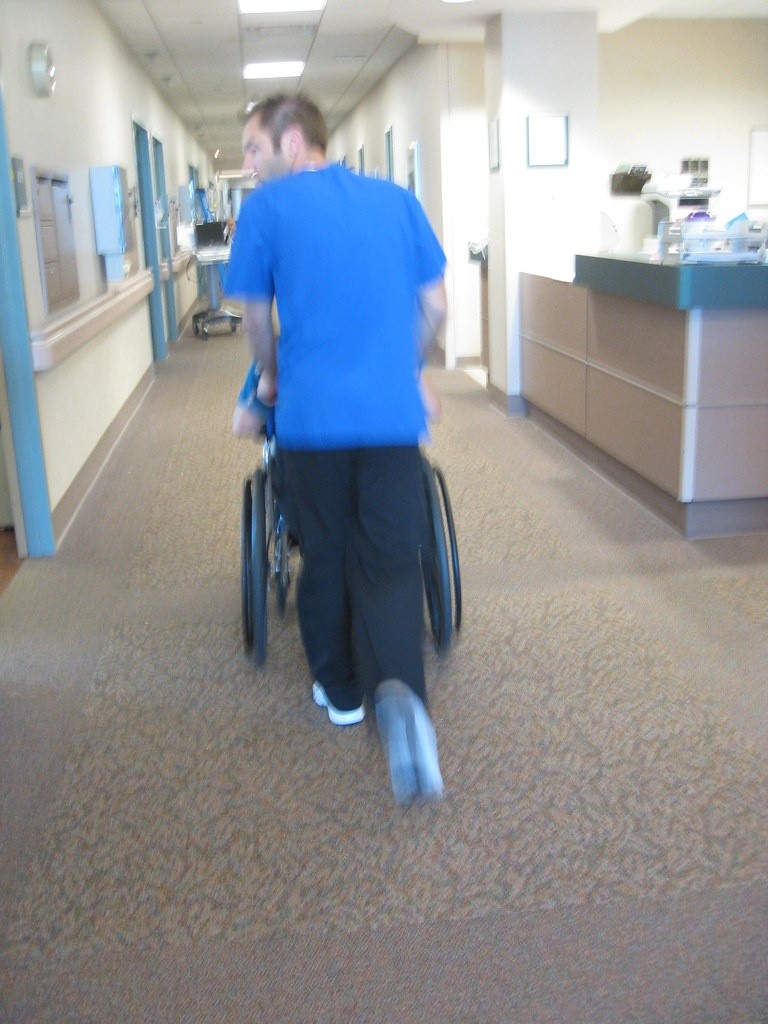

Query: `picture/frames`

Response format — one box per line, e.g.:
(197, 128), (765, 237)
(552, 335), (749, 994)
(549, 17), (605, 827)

(488, 117), (499, 169)
(527, 113), (568, 167)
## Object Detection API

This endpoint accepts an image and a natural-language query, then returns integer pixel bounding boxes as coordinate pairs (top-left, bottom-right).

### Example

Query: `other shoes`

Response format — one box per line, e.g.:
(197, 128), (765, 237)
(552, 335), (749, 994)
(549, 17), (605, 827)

(373, 677), (444, 804)
(312, 680), (366, 726)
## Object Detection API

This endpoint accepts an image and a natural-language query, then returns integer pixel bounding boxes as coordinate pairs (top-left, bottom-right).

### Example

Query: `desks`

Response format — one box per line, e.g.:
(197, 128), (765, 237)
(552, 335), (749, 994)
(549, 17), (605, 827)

(190, 246), (243, 339)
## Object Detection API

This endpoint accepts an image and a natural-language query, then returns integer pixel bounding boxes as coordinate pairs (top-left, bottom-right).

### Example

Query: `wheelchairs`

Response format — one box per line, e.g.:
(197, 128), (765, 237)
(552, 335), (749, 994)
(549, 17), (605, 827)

(240, 379), (464, 667)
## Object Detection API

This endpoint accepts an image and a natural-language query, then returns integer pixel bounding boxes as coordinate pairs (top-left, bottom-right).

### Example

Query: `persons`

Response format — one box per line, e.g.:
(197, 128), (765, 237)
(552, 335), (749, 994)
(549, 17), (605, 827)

(220, 88), (449, 807)
